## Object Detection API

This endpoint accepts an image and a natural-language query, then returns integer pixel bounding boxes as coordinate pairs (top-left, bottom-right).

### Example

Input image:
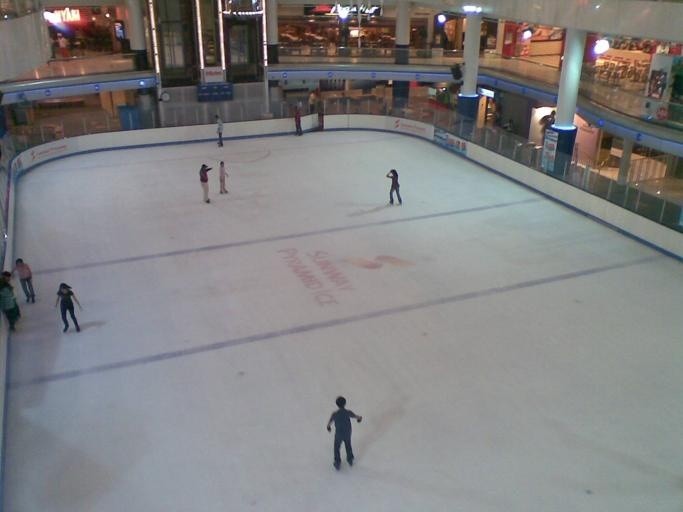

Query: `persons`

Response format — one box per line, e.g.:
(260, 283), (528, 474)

(327, 18), (350, 46)
(56, 282), (81, 332)
(199, 164), (212, 203)
(387, 169), (402, 204)
(215, 115), (223, 147)
(57, 20), (114, 57)
(218, 161), (228, 194)
(540, 109), (556, 146)
(480, 29), (489, 57)
(326, 396), (362, 469)
(0, 258), (35, 330)
(288, 90), (325, 134)
(489, 97), (514, 132)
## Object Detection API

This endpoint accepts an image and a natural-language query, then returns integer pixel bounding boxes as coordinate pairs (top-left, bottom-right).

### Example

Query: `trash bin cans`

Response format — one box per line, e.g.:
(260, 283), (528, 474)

(119, 105), (140, 130)
